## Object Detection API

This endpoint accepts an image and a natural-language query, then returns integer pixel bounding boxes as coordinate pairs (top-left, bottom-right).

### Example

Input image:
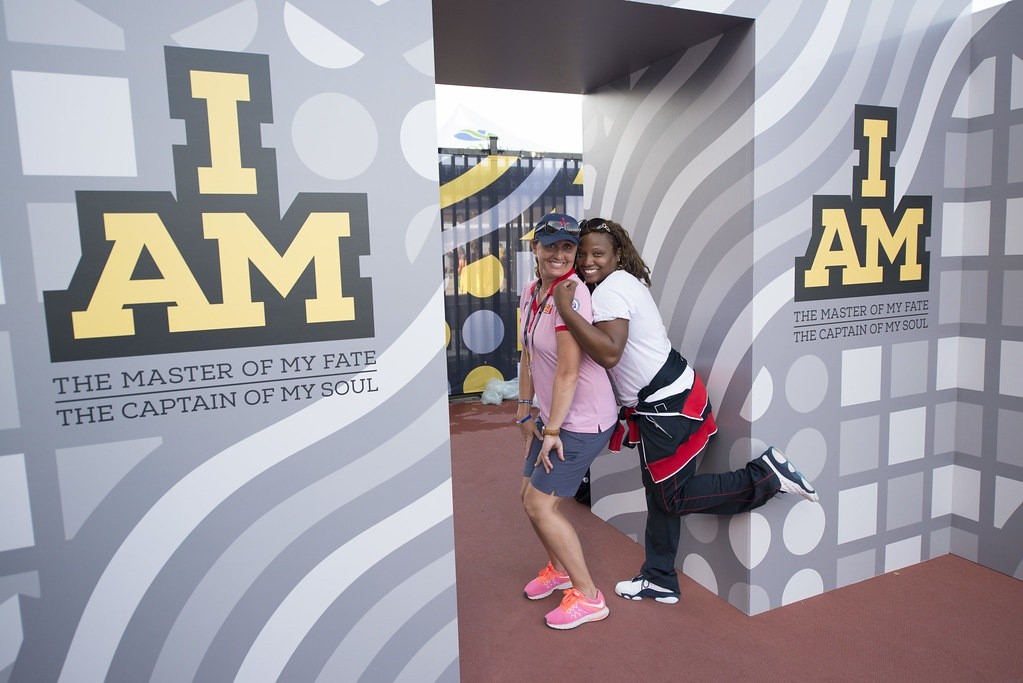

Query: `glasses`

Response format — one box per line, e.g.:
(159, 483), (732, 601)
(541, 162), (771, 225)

(579, 219), (614, 235)
(535, 221), (582, 237)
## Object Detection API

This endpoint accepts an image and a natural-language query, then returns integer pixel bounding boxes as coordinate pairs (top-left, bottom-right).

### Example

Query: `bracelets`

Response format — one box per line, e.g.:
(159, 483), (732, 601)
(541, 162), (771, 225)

(518, 399), (534, 405)
(516, 414), (531, 425)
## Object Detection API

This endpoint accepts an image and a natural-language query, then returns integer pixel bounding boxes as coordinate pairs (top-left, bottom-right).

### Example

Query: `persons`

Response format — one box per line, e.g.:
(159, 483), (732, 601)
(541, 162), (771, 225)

(553, 218), (819, 604)
(443, 212), (505, 276)
(518, 212), (619, 631)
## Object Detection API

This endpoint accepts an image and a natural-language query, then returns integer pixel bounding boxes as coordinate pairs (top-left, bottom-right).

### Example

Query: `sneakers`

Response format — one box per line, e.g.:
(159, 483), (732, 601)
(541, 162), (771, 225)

(615, 574), (679, 604)
(759, 446), (819, 502)
(524, 560), (575, 600)
(545, 587), (610, 630)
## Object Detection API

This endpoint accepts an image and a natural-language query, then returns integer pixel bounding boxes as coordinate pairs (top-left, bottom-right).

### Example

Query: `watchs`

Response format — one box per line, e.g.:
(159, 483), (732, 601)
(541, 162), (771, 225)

(542, 425), (560, 437)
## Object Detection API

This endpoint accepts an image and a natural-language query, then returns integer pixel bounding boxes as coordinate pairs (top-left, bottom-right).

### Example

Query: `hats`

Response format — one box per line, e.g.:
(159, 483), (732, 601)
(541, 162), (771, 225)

(534, 214), (580, 246)
